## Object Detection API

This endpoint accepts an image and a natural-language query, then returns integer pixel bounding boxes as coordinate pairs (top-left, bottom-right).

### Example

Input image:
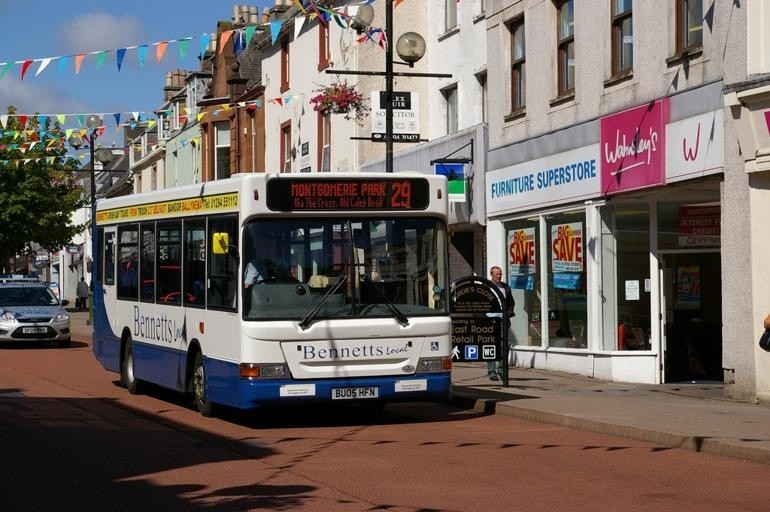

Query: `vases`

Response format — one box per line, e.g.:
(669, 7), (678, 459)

(332, 106), (348, 113)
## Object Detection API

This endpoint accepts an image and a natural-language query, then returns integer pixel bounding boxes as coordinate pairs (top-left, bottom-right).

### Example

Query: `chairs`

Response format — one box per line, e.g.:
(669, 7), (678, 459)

(121, 260), (204, 304)
(567, 320), (586, 347)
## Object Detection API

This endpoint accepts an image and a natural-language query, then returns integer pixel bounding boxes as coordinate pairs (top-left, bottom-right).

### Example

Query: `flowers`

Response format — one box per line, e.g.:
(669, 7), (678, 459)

(309, 77), (372, 127)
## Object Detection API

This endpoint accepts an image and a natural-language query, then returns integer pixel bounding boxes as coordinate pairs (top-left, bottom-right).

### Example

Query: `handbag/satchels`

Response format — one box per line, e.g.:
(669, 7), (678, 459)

(760, 328), (770, 352)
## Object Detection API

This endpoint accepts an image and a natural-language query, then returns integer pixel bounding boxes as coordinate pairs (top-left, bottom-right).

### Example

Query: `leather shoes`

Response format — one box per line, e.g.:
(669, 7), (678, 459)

(497, 367), (503, 378)
(488, 371), (498, 380)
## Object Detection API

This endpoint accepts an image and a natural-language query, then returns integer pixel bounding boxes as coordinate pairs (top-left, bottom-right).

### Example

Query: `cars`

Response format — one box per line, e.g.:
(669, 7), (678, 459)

(0, 278), (71, 342)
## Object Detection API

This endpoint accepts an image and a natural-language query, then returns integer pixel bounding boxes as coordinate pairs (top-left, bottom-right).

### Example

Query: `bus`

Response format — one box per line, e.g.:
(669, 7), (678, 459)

(90, 172), (451, 417)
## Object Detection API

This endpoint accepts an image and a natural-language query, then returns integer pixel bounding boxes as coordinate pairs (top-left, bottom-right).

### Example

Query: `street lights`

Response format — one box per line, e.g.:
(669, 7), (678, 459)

(343, 1), (426, 173)
(70, 114), (103, 204)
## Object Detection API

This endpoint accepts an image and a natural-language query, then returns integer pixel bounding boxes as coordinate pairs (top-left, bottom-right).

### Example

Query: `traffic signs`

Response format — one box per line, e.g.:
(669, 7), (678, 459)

(451, 317), (503, 362)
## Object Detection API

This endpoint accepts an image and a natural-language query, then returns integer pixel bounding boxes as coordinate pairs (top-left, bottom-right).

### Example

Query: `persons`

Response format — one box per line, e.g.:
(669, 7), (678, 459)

(77, 277), (88, 312)
(243, 239), (291, 288)
(488, 267), (516, 381)
(120, 260), (137, 286)
